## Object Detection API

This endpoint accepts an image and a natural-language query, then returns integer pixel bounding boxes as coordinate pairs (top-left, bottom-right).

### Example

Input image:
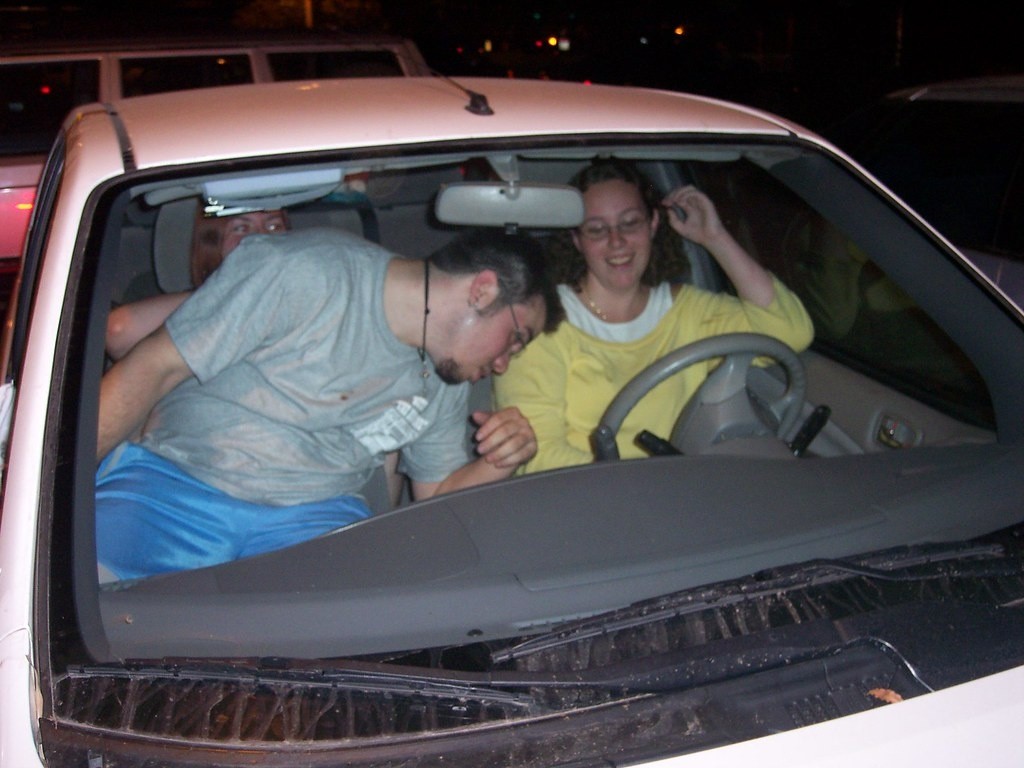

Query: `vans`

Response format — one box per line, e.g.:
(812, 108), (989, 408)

(0, 29), (435, 312)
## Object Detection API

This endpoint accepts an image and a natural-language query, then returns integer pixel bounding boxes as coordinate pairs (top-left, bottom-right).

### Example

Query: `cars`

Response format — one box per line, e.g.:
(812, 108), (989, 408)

(798, 76), (1024, 314)
(1, 75), (1024, 768)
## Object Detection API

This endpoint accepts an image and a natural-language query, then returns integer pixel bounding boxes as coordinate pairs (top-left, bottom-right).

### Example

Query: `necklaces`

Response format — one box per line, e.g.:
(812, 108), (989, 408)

(577, 282), (607, 321)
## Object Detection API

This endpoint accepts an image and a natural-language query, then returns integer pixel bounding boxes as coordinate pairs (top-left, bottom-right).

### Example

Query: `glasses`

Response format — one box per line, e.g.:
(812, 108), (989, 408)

(575, 208), (653, 240)
(500, 278), (528, 357)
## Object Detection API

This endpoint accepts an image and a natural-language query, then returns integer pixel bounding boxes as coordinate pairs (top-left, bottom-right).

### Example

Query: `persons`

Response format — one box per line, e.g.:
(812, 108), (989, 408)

(768, 209), (954, 376)
(97, 225), (569, 595)
(104, 198), (296, 366)
(487, 157), (816, 477)
